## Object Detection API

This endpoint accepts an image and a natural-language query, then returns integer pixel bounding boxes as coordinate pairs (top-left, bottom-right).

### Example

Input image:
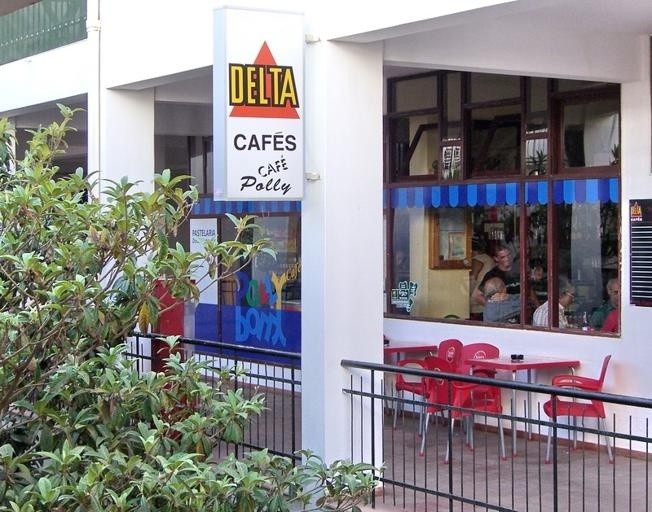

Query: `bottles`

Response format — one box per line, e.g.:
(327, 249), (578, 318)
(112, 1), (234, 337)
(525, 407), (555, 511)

(564, 306), (581, 324)
(489, 227), (505, 240)
(580, 309), (599, 331)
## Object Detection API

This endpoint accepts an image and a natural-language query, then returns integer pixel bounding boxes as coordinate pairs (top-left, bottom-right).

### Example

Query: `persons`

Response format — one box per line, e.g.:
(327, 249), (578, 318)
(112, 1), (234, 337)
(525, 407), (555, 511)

(469, 240), (620, 332)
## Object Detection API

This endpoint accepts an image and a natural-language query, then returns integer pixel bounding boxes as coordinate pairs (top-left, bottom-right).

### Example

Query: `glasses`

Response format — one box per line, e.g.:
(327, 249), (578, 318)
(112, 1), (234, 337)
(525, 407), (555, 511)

(610, 289), (617, 295)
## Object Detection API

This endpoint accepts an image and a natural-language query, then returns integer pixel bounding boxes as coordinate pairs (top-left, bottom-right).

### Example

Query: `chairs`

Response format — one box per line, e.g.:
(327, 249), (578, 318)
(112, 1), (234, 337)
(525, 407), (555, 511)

(384, 338), (613, 466)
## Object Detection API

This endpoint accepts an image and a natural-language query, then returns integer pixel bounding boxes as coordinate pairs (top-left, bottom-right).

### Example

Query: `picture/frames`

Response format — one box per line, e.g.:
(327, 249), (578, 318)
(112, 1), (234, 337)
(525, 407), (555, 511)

(429, 205), (474, 271)
